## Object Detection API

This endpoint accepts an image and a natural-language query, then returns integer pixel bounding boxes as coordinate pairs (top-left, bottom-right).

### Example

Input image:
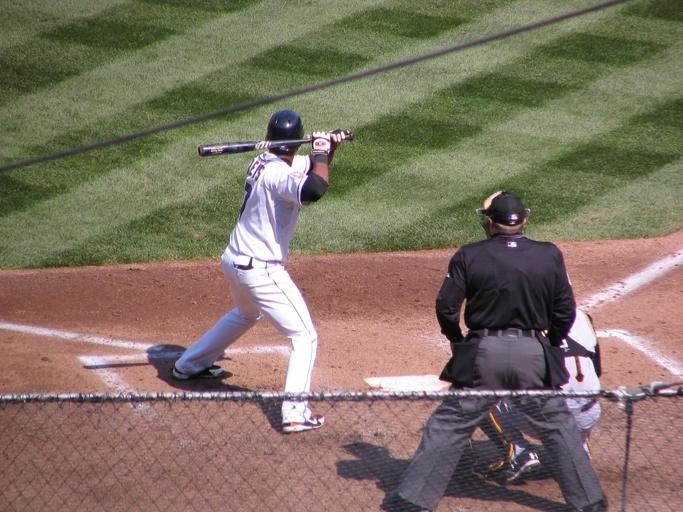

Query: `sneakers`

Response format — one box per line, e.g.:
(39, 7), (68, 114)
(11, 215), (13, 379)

(505, 449), (540, 480)
(282, 414), (325, 433)
(392, 492), (431, 512)
(172, 366), (226, 379)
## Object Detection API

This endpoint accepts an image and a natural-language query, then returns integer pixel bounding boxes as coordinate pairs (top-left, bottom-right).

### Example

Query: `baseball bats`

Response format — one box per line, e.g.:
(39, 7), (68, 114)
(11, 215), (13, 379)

(197, 133), (355, 156)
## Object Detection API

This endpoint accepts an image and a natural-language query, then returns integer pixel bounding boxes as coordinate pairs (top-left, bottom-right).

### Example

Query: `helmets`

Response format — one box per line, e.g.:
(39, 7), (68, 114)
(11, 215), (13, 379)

(476, 190), (530, 226)
(265, 110), (305, 152)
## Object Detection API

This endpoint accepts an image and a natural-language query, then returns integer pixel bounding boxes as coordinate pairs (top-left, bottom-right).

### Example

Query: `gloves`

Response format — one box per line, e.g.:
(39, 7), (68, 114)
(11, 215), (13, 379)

(309, 131), (331, 156)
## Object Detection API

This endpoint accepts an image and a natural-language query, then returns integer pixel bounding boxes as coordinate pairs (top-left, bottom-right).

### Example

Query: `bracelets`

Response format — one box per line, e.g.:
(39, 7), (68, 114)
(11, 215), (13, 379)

(311, 154), (329, 167)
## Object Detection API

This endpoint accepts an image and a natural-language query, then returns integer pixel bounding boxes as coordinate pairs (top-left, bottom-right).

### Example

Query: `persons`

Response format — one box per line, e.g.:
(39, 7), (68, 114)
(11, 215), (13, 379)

(470, 308), (604, 486)
(383, 189), (611, 511)
(169, 109), (351, 434)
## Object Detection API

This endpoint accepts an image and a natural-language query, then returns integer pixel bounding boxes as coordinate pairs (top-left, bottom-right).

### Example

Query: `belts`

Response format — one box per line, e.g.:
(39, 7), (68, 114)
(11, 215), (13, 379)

(582, 398), (595, 411)
(471, 329), (536, 338)
